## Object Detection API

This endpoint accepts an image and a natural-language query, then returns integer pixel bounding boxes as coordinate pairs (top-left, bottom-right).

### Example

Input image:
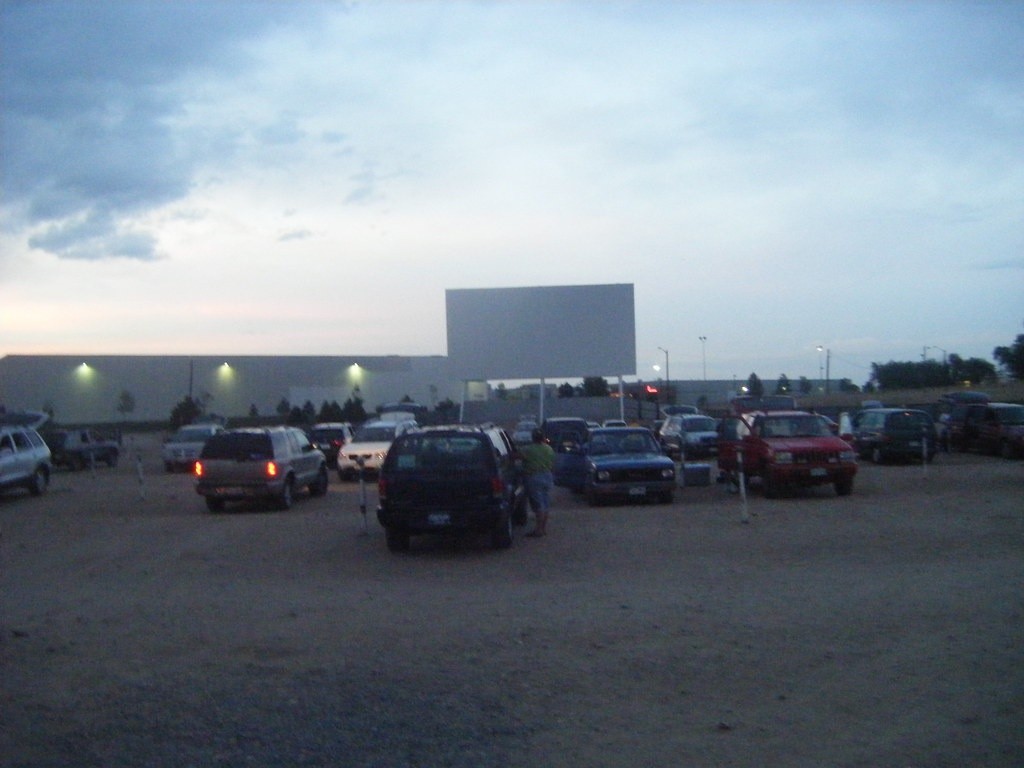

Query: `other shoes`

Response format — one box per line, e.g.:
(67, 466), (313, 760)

(525, 529), (547, 537)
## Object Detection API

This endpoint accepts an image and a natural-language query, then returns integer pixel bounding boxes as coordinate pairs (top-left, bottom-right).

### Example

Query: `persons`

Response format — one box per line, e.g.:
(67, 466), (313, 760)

(513, 428), (554, 536)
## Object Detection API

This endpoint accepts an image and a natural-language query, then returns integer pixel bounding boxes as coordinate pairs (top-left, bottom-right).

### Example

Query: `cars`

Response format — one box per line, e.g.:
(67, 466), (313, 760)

(336, 419), (428, 481)
(551, 426), (676, 505)
(48, 429), (120, 471)
(658, 403), (719, 453)
(309, 420), (356, 464)
(941, 401), (1024, 459)
(513, 416), (630, 471)
(160, 424), (227, 473)
(840, 406), (939, 465)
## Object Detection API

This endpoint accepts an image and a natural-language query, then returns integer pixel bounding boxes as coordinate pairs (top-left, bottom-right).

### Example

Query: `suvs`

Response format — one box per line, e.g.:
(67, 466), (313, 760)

(375, 421), (530, 549)
(716, 396), (860, 498)
(0, 423), (53, 494)
(192, 424), (329, 514)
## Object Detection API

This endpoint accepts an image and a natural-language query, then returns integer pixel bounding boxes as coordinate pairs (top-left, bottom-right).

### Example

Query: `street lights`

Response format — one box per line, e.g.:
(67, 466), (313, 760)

(699, 336), (706, 394)
(817, 346), (830, 395)
(657, 346), (670, 400)
(932, 343), (947, 364)
